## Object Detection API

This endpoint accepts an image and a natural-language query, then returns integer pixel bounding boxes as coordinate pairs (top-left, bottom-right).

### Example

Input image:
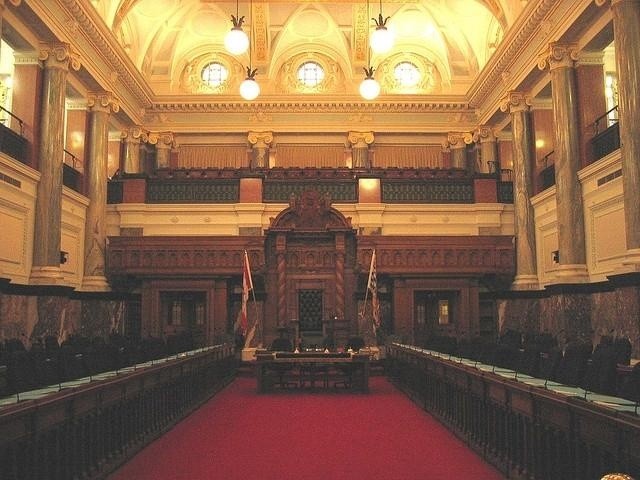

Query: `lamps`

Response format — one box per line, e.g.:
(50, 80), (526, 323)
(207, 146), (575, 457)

(360, 47), (380, 99)
(239, 30), (261, 99)
(226, 0), (248, 55)
(370, 0), (394, 52)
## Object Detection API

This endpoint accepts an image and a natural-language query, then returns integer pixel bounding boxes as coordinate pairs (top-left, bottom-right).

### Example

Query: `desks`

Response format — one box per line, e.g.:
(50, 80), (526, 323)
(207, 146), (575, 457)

(255, 349), (384, 395)
(388, 342), (640, 480)
(1, 342), (241, 480)
(424, 330), (640, 404)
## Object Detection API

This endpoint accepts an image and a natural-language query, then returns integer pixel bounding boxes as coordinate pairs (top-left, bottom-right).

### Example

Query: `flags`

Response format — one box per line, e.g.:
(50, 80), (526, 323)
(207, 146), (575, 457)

(240, 253), (254, 338)
(367, 253), (381, 330)
